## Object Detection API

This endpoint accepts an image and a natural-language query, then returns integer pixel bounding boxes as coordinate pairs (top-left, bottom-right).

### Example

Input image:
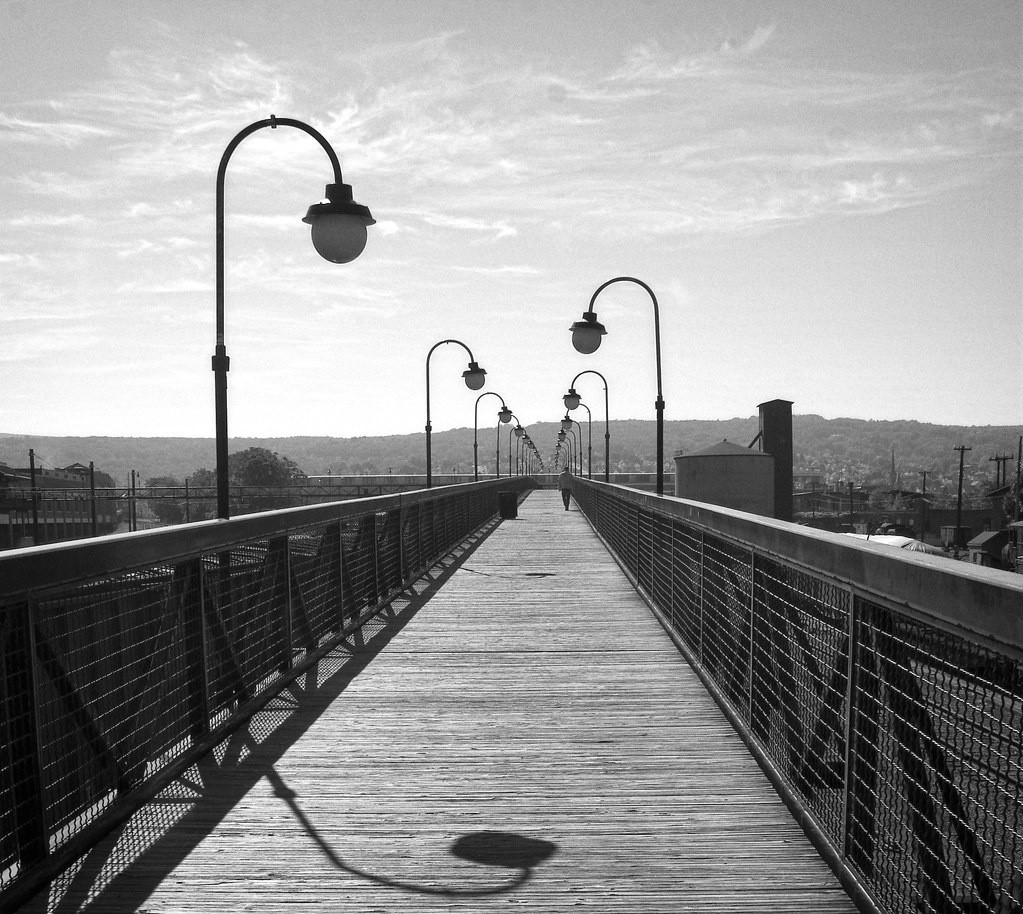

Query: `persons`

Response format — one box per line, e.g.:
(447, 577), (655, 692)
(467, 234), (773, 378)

(557, 467), (573, 511)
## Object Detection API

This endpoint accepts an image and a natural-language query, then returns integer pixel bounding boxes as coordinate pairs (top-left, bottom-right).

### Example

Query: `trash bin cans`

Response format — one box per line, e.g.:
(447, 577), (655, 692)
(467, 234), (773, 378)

(499, 490), (518, 520)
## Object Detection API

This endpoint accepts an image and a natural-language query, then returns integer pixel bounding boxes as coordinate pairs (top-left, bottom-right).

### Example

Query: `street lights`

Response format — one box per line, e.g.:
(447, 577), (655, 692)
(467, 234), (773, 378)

(473, 370), (612, 483)
(426, 340), (487, 489)
(212, 115), (376, 715)
(568, 277), (665, 497)
